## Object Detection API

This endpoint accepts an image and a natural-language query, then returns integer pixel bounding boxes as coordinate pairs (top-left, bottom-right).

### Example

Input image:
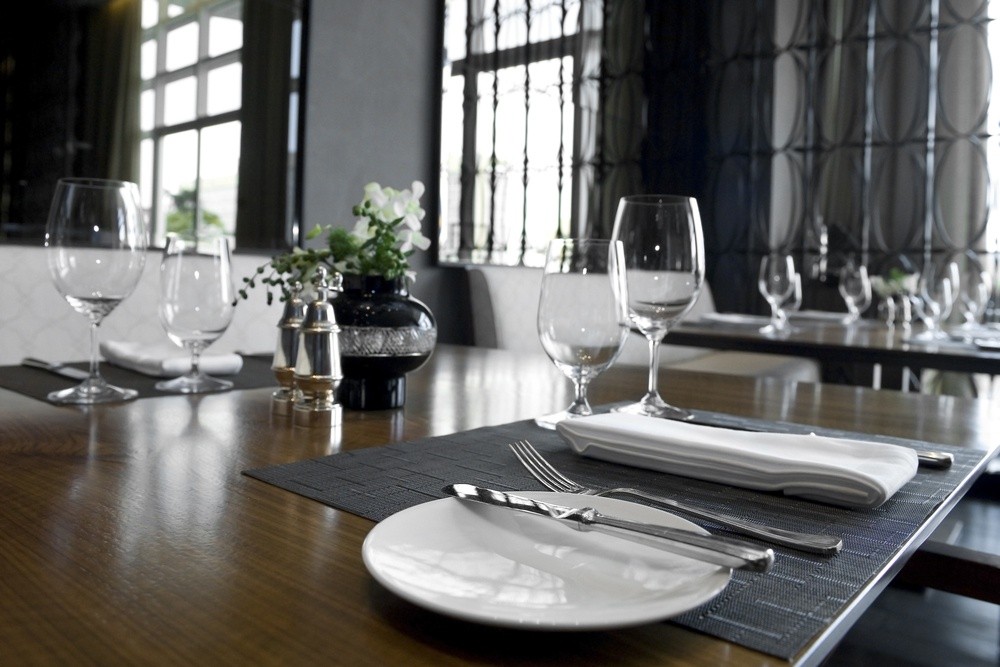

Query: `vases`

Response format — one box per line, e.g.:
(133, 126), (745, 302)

(283, 271), (438, 408)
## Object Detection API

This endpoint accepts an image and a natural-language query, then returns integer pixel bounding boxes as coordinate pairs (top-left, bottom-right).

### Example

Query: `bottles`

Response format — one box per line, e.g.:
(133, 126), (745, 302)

(293, 290), (345, 426)
(271, 292), (307, 416)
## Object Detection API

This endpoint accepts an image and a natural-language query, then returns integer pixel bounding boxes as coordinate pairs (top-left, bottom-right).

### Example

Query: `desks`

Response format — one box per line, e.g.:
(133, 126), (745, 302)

(0, 341), (999, 667)
(634, 311), (1000, 603)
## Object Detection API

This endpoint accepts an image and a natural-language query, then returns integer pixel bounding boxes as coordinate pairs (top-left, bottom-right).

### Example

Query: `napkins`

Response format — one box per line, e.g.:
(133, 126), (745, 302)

(550, 407), (922, 517)
(98, 338), (248, 379)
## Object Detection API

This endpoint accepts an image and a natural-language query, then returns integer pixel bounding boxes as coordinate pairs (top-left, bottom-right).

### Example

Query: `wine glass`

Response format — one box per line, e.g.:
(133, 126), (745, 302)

(45, 178), (148, 405)
(155, 233), (237, 392)
(840, 249), (1000, 344)
(774, 273), (801, 334)
(607, 196), (707, 421)
(535, 237), (632, 430)
(758, 252), (795, 340)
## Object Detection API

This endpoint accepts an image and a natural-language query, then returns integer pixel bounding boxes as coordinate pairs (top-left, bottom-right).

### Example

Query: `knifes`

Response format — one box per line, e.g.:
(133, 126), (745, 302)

(507, 438), (843, 554)
(663, 414), (954, 470)
(22, 357), (104, 382)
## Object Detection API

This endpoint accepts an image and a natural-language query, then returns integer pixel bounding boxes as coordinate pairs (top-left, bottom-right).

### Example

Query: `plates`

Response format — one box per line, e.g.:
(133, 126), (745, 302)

(975, 340), (1000, 349)
(362, 491), (734, 629)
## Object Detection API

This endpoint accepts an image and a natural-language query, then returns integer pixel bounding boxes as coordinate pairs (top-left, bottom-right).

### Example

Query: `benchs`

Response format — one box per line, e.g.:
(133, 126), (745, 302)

(0, 223), (716, 364)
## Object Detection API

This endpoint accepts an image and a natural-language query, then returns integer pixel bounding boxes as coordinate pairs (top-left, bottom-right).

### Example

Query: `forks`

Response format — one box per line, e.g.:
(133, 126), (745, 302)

(442, 483), (775, 573)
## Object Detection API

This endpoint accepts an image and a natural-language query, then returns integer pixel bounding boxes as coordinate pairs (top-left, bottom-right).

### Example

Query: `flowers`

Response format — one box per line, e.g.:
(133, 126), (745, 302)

(310, 180), (436, 278)
